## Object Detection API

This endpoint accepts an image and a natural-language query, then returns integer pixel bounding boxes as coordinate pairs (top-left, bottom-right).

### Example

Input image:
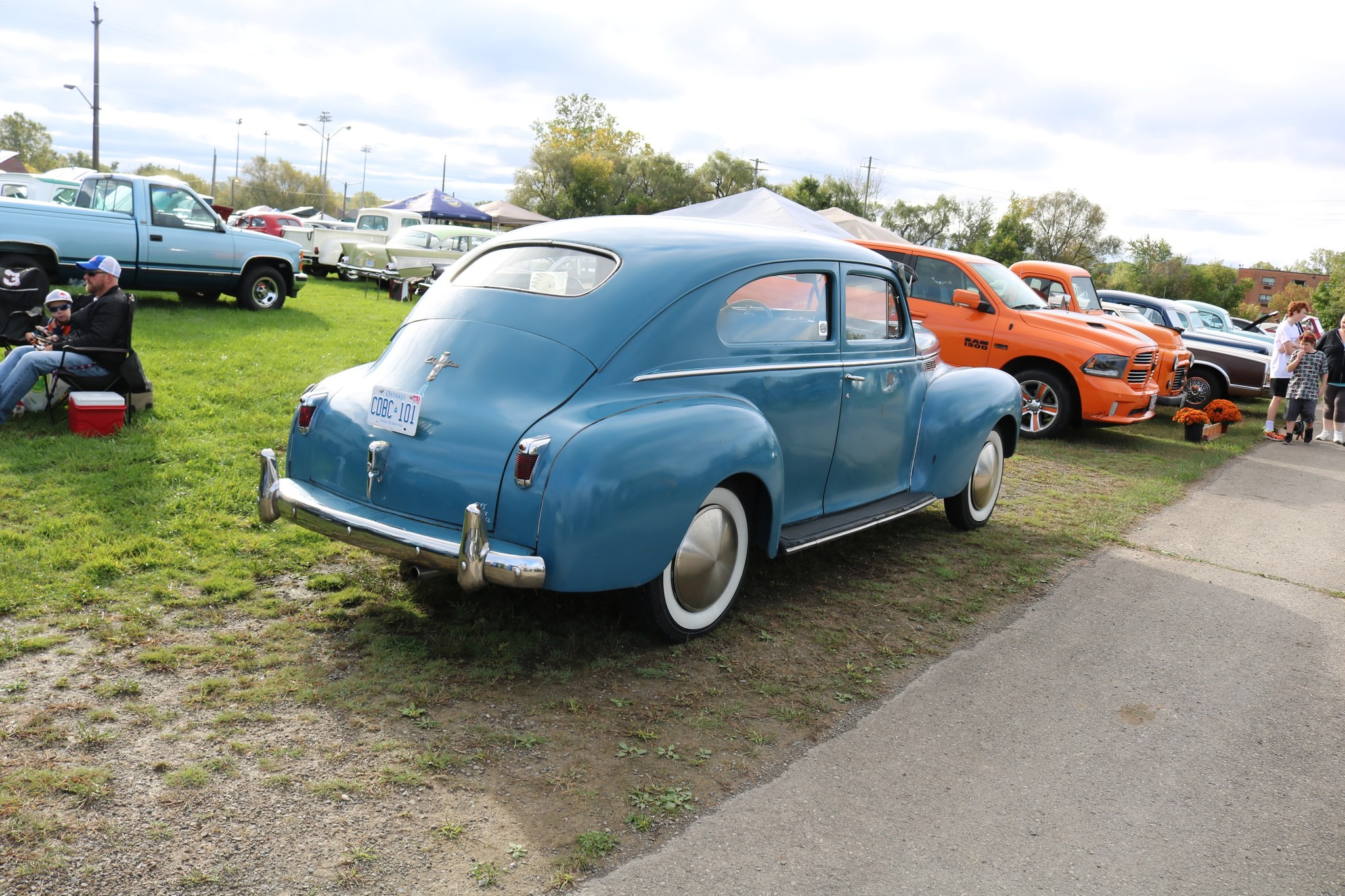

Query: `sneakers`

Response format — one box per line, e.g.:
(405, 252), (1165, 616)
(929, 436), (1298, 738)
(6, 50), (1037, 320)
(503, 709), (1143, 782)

(1316, 430), (1331, 440)
(1262, 429), (1284, 441)
(1333, 431), (1343, 444)
(1262, 426), (1286, 438)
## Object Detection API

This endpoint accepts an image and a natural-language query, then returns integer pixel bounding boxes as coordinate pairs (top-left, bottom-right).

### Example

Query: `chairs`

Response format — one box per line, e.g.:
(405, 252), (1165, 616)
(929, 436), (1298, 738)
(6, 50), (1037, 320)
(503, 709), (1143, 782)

(934, 264), (957, 305)
(911, 259), (941, 302)
(33, 294), (138, 431)
(0, 267), (47, 358)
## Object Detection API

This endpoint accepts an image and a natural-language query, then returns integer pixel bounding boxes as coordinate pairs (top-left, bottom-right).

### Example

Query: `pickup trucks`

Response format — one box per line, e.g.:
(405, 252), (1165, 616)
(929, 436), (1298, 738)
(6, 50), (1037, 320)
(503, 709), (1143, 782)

(0, 172), (307, 314)
(281, 207), (466, 283)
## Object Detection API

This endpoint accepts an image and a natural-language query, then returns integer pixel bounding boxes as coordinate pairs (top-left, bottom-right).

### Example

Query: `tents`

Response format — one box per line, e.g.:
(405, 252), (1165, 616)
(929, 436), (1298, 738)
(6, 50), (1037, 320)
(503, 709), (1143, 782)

(377, 188), (499, 231)
(814, 207), (914, 244)
(474, 201), (557, 230)
(650, 187), (859, 240)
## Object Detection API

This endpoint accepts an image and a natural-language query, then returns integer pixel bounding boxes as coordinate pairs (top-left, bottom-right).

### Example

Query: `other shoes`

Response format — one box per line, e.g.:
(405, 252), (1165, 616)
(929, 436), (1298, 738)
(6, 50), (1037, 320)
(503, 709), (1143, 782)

(1304, 427), (1314, 444)
(1281, 433), (1293, 444)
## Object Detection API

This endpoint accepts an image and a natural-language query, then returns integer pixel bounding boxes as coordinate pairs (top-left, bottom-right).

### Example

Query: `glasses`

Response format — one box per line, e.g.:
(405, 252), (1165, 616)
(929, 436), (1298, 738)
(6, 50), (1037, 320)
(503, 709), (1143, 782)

(1299, 335), (1313, 340)
(83, 270), (109, 277)
(49, 304), (71, 312)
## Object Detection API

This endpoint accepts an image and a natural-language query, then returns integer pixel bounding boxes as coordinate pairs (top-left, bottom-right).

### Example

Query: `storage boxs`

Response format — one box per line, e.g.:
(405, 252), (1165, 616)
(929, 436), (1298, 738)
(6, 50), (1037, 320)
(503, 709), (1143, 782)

(69, 391), (127, 436)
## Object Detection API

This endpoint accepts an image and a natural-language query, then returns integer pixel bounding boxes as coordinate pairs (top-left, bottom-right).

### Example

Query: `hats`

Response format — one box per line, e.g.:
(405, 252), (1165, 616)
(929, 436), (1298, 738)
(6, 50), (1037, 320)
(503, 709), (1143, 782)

(44, 289), (73, 309)
(75, 254), (122, 278)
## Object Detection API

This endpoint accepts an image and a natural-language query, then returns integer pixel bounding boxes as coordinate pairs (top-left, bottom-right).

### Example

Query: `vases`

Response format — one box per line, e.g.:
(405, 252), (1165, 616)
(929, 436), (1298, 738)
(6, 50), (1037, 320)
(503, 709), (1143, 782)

(1184, 421), (1203, 441)
(1212, 421), (1228, 433)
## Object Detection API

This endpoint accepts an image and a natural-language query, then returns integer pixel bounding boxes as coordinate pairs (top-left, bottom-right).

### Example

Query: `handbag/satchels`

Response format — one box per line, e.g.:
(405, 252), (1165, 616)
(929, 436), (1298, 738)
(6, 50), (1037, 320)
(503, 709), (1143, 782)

(21, 373), (71, 413)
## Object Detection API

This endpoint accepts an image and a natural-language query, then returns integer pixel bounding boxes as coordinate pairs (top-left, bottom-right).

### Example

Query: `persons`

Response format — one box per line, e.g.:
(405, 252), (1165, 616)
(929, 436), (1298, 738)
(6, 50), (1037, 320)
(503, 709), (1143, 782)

(1313, 313), (1345, 443)
(1281, 330), (1329, 445)
(1261, 300), (1310, 441)
(0, 255), (129, 427)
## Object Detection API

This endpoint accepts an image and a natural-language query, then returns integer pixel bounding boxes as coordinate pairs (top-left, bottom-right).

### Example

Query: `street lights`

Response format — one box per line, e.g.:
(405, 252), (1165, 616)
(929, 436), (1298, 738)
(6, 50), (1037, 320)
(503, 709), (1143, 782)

(316, 111), (333, 208)
(297, 124), (351, 219)
(64, 84), (100, 174)
(360, 144), (373, 208)
(330, 177), (362, 221)
(263, 131), (271, 181)
(235, 118), (243, 178)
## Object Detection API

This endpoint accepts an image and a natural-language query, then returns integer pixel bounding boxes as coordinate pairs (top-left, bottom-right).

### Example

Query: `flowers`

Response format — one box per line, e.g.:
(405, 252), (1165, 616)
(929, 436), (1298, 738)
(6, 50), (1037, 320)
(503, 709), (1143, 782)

(1172, 407), (1210, 424)
(1204, 399), (1243, 425)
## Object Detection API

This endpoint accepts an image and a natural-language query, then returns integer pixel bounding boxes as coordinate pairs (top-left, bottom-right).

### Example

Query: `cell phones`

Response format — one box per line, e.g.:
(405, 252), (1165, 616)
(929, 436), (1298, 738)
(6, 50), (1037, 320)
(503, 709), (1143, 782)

(32, 333), (56, 344)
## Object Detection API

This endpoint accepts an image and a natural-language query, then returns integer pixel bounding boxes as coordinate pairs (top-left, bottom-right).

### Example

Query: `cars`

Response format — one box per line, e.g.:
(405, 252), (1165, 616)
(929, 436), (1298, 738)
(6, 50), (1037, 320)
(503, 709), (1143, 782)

(252, 214), (1021, 643)
(1, 150), (371, 238)
(337, 224), (599, 296)
(728, 240), (1324, 441)
